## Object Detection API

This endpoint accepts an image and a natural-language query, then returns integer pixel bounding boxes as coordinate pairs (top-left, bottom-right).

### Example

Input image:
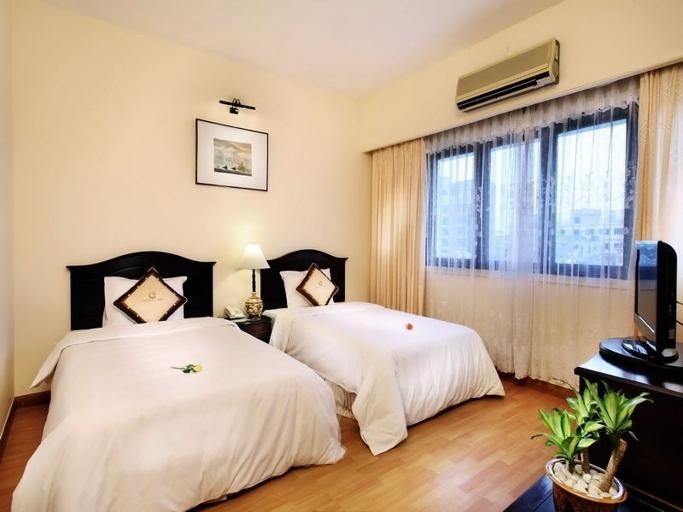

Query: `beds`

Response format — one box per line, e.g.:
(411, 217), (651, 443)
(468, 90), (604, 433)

(261, 250), (506, 457)
(11, 251), (346, 512)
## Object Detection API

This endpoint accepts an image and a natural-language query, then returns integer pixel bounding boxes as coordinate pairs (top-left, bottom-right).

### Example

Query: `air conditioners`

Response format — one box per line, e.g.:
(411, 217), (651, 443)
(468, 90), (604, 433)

(455, 39), (560, 113)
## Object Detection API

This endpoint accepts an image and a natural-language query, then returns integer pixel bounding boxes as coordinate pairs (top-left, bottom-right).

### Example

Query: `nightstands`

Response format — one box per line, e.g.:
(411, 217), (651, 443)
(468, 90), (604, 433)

(226, 315), (272, 343)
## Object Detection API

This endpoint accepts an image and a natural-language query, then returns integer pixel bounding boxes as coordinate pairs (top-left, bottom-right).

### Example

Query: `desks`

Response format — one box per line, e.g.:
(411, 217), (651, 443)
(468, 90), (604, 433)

(504, 473), (649, 512)
(574, 352), (683, 512)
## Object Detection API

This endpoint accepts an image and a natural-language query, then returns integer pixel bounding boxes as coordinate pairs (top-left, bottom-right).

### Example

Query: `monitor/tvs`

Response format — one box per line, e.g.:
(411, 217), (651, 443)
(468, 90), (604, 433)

(622, 239), (680, 365)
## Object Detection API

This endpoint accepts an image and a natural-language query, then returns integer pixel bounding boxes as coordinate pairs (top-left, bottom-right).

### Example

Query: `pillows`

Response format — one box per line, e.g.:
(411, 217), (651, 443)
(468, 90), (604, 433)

(100, 276), (188, 326)
(113, 266), (188, 324)
(296, 263), (340, 306)
(279, 268), (334, 308)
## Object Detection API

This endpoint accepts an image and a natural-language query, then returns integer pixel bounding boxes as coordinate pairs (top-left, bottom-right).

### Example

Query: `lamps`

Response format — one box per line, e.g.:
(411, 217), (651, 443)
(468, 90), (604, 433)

(236, 244), (271, 319)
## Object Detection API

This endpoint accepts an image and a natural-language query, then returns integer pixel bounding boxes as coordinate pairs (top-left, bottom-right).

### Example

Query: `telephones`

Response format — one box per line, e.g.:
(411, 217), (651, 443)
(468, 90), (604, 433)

(224, 305), (246, 318)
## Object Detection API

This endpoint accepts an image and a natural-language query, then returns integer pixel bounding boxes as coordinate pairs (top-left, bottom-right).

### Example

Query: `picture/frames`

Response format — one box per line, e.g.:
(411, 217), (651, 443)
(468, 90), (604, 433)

(196, 118), (268, 191)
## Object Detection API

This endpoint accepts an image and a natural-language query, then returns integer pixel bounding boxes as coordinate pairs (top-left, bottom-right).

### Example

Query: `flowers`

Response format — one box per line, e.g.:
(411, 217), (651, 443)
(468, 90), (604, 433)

(171, 364), (204, 372)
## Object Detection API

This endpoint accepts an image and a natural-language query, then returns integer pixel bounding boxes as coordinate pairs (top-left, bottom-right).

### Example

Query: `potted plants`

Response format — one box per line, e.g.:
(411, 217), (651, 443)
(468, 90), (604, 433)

(529, 375), (654, 512)
